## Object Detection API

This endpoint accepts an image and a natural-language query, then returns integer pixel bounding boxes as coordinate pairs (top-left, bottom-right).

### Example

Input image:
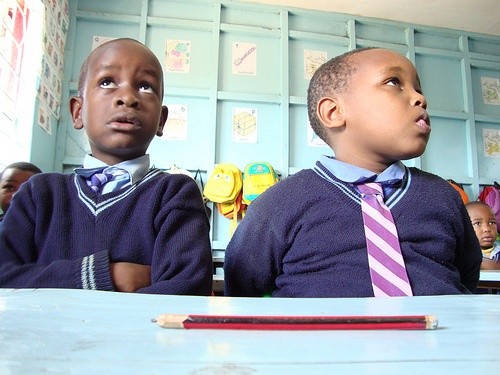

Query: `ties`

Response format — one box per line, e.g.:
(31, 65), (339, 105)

(87, 171), (114, 196)
(355, 182), (415, 297)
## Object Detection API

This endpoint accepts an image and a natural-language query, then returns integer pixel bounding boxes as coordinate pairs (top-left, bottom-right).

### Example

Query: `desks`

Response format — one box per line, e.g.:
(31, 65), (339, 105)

(0, 256), (500, 375)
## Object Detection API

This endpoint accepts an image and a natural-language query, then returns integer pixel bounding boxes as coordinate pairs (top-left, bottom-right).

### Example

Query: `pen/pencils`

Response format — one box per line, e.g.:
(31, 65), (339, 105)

(151, 314), (437, 331)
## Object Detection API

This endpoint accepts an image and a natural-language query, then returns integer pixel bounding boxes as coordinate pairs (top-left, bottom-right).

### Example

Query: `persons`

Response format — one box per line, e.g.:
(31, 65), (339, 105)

(465, 201), (500, 270)
(224, 49), (483, 296)
(0, 163), (44, 223)
(0, 38), (214, 297)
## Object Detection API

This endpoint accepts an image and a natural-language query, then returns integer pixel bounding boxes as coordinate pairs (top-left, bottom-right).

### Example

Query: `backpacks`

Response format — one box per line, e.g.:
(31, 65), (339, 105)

(216, 191), (246, 220)
(202, 162), (242, 205)
(485, 187), (500, 230)
(243, 162), (280, 205)
